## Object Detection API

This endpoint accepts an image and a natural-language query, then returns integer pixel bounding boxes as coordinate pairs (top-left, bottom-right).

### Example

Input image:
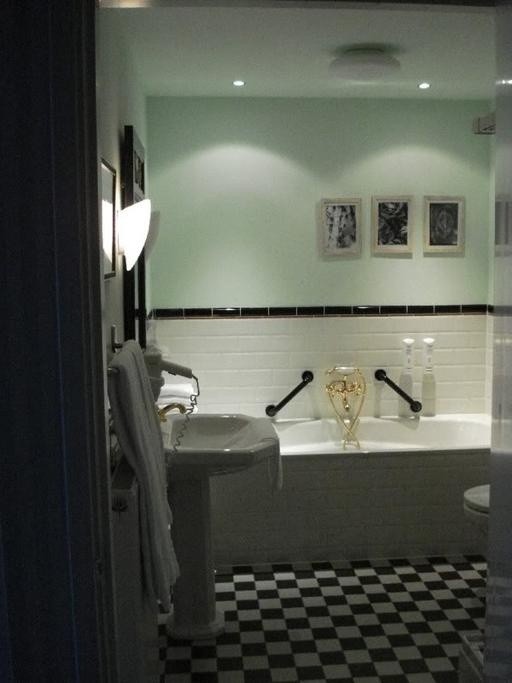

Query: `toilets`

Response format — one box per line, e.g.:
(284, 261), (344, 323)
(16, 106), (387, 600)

(462, 483), (491, 525)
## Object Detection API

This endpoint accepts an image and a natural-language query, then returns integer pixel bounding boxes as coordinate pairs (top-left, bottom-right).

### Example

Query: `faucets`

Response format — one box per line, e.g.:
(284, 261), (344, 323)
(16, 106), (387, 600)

(156, 403), (186, 423)
(340, 398), (351, 413)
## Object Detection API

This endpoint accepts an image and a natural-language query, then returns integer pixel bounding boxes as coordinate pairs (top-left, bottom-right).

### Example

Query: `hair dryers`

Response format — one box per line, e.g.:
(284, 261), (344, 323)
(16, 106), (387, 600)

(141, 350), (194, 401)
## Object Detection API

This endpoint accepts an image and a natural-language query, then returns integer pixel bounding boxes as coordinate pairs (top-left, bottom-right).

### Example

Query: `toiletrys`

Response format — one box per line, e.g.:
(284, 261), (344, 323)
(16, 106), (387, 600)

(421, 337), (438, 418)
(397, 339), (418, 413)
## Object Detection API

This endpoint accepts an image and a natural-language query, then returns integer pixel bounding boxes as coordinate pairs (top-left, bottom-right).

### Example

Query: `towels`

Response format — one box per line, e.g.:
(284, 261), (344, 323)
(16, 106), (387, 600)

(107, 338), (181, 611)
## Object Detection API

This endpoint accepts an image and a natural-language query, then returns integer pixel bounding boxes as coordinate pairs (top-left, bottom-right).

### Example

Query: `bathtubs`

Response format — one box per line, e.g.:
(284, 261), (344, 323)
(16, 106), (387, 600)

(275, 411), (495, 458)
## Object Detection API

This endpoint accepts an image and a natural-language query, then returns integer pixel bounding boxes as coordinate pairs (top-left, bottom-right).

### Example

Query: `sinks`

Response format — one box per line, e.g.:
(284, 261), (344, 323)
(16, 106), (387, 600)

(158, 413), (275, 478)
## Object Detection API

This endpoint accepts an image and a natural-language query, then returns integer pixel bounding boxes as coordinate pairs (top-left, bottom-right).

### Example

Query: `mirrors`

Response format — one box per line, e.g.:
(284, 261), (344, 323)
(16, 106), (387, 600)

(98, 158), (116, 279)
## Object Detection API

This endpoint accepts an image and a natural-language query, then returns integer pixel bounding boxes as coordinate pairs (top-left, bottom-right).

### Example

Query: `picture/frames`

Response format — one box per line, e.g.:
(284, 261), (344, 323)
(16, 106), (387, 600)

(318, 196), (463, 255)
